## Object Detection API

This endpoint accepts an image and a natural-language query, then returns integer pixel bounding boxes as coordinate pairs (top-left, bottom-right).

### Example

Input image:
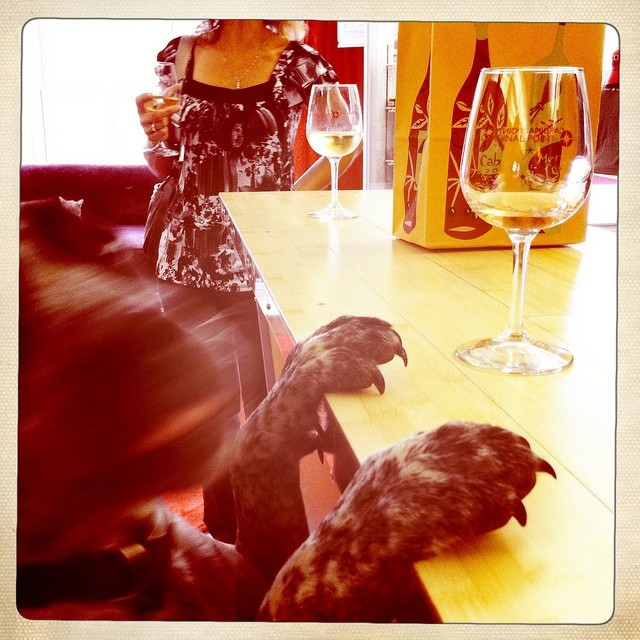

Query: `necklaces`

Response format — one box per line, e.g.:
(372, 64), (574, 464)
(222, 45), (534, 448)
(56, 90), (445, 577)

(217, 37), (267, 89)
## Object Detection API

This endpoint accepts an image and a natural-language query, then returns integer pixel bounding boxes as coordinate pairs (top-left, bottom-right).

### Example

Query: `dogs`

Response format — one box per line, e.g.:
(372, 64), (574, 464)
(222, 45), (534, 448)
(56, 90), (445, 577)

(18, 201), (556, 624)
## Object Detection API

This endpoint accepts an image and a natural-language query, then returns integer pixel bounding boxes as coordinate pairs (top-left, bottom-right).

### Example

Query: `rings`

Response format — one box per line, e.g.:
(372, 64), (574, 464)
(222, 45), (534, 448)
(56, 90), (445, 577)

(151, 123), (156, 132)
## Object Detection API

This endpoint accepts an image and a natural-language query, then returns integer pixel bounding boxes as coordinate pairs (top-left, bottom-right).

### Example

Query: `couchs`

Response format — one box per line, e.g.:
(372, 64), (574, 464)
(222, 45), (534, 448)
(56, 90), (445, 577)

(21, 164), (160, 250)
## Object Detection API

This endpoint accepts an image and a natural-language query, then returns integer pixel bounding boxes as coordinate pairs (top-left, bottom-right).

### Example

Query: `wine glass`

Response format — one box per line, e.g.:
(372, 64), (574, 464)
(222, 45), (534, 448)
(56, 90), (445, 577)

(453, 65), (594, 378)
(140, 62), (180, 158)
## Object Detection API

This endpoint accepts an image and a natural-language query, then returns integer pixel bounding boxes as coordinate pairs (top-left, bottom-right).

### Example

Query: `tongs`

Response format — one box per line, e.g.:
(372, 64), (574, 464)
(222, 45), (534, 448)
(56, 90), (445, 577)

(305, 83), (363, 221)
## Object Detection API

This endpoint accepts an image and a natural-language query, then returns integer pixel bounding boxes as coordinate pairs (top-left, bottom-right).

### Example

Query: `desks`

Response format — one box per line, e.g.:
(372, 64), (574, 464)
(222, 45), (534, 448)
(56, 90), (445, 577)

(215, 188), (617, 621)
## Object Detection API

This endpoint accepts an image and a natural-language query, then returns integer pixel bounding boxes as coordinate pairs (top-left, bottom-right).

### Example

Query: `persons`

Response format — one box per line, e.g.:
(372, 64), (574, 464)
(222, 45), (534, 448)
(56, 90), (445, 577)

(134, 21), (365, 544)
